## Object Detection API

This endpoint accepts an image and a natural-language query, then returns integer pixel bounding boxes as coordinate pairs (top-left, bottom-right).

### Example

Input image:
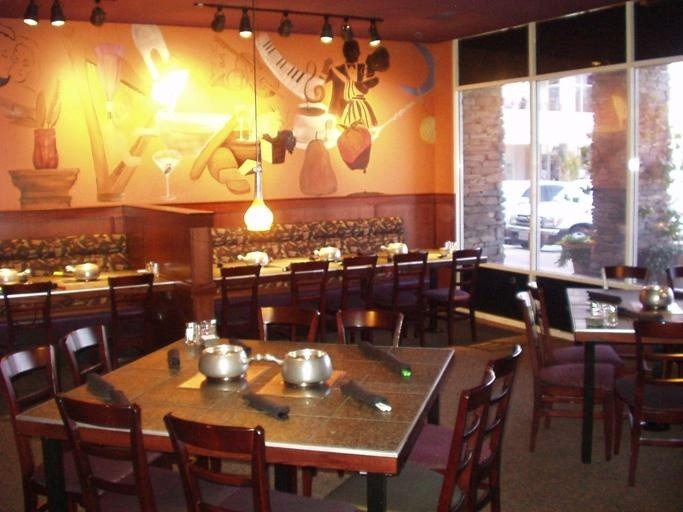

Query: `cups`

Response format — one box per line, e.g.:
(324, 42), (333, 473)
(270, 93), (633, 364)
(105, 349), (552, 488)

(293, 102), (337, 142)
(146, 263), (160, 283)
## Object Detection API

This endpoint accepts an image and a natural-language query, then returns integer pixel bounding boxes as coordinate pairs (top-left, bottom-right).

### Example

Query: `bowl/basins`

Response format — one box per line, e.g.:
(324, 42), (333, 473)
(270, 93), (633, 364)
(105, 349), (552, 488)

(8, 167), (79, 209)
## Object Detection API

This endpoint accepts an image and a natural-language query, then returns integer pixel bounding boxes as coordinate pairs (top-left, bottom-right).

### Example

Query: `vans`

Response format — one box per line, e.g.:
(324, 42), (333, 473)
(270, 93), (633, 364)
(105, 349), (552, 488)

(505, 180), (592, 249)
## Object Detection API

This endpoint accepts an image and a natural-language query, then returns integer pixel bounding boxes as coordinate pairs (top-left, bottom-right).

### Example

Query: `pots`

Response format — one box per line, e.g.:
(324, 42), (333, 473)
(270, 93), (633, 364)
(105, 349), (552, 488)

(64, 262), (101, 282)
(0, 266), (31, 286)
(313, 246), (341, 263)
(638, 281), (674, 312)
(263, 349), (333, 388)
(237, 250), (271, 268)
(379, 242), (408, 260)
(199, 345), (263, 383)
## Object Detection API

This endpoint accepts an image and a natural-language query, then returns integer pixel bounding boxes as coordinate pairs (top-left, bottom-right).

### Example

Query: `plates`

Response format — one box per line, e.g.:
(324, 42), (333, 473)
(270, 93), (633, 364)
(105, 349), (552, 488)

(289, 130), (342, 150)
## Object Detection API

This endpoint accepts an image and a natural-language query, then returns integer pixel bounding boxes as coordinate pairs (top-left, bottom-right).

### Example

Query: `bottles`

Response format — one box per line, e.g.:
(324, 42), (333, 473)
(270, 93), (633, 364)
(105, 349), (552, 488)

(224, 130), (261, 175)
(183, 318), (218, 347)
(439, 240), (458, 259)
(588, 298), (620, 330)
(29, 126), (59, 169)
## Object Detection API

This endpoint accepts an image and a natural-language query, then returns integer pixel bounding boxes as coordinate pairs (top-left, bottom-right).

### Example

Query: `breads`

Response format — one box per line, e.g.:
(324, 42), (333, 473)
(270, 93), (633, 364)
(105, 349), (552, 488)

(189, 115), (250, 193)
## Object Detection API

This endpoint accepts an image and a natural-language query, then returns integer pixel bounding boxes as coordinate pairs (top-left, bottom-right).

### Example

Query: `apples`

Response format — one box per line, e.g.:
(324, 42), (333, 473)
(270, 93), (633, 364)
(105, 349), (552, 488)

(337, 120), (372, 170)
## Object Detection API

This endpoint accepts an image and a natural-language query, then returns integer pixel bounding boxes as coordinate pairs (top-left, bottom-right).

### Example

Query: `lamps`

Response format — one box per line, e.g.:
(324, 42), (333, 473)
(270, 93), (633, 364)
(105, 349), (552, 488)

(22, 0), (106, 31)
(243, 4), (274, 235)
(210, 7), (382, 47)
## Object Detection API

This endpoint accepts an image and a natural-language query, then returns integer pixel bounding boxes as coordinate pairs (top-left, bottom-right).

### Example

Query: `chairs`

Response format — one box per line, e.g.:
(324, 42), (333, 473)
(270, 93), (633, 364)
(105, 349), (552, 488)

(515, 263), (682, 490)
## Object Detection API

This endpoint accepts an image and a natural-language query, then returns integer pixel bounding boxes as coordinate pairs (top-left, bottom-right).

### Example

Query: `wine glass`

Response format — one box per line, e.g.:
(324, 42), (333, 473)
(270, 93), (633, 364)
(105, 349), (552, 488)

(151, 147), (183, 204)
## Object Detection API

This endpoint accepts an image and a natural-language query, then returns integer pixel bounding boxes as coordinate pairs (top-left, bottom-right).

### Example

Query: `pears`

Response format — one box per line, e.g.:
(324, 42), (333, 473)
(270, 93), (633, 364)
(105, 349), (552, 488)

(299, 134), (336, 196)
